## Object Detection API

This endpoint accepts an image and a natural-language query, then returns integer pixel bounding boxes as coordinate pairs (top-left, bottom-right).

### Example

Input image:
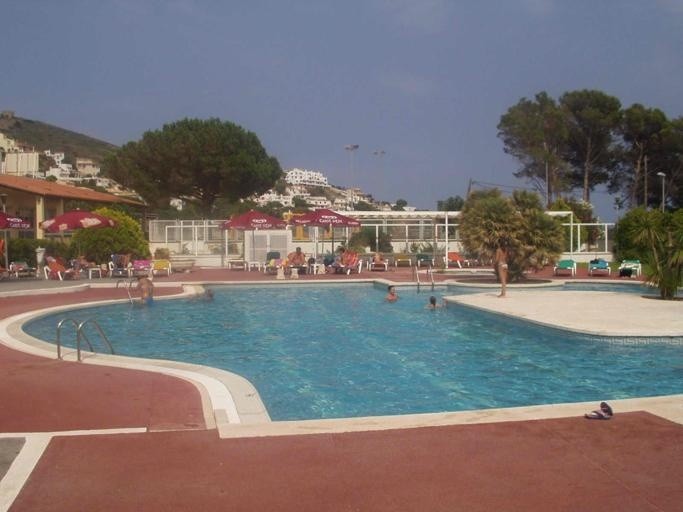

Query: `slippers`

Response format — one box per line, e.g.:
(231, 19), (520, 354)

(585, 401), (613, 420)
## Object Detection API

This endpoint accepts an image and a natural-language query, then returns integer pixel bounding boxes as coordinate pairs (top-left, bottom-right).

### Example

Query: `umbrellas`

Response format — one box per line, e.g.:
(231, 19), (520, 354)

(37, 207), (119, 279)
(216, 209), (288, 272)
(285, 208), (361, 272)
(0, 212), (31, 278)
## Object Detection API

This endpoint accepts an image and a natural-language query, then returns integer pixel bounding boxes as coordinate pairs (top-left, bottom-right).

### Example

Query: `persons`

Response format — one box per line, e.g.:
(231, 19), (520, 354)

(382, 285), (402, 303)
(286, 247), (304, 266)
(130, 273), (153, 305)
(331, 247), (349, 268)
(422, 295), (448, 309)
(490, 238), (509, 299)
(72, 251), (95, 273)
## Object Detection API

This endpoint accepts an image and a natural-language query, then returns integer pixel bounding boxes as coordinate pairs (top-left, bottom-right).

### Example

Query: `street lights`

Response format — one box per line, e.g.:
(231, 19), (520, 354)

(373, 148), (387, 211)
(344, 143), (358, 210)
(656, 170), (667, 213)
